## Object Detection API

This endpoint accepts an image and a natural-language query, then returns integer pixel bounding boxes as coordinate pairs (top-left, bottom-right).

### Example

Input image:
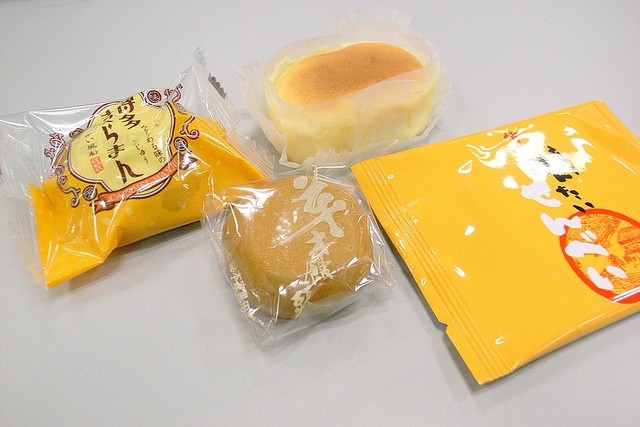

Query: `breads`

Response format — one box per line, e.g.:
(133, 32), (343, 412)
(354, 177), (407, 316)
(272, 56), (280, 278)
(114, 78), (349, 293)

(218, 176), (371, 320)
(247, 39), (436, 166)
